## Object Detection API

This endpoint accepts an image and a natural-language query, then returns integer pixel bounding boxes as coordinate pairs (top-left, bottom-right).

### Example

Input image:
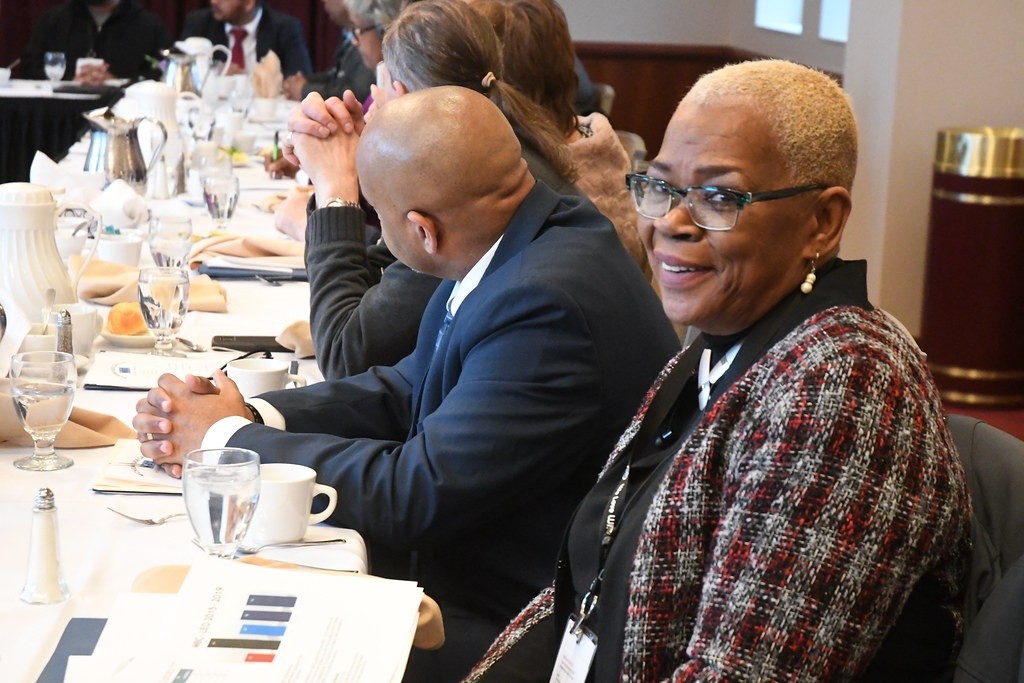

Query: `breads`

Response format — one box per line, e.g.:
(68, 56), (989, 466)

(106, 300), (149, 334)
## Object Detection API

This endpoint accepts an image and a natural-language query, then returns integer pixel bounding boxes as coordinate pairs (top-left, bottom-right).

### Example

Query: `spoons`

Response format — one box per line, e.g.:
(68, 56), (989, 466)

(236, 538), (346, 553)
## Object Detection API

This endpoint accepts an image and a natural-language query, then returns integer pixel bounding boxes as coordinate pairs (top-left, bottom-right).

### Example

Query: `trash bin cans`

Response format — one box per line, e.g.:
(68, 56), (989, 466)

(920, 127), (1024, 411)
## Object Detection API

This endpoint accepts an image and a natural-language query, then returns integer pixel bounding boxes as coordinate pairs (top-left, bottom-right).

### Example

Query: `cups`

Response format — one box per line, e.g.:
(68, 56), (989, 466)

(44, 304), (103, 357)
(22, 325), (57, 363)
(227, 358), (307, 397)
(54, 215), (144, 269)
(182, 72), (258, 229)
(238, 462), (338, 544)
(0, 68), (12, 86)
(148, 215), (193, 266)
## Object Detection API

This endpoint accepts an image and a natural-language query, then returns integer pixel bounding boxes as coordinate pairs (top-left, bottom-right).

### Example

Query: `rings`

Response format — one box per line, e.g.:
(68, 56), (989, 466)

(147, 433), (153, 441)
(287, 132), (294, 143)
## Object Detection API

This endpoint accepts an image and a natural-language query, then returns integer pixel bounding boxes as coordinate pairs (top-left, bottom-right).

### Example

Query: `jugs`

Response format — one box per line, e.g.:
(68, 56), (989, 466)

(123, 35), (232, 197)
(0, 182), (103, 374)
(82, 107), (168, 202)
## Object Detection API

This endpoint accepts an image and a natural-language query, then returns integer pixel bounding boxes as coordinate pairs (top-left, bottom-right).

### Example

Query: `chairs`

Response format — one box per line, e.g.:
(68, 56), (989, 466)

(948, 413), (1024, 683)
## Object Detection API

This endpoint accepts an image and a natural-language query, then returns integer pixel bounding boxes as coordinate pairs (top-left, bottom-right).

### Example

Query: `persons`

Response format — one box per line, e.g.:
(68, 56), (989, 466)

(275, 0), (655, 282)
(135, 85), (680, 682)
(463, 56), (969, 683)
(265, 0), (402, 180)
(279, 0), (578, 381)
(179, 0), (311, 78)
(22, 0), (173, 80)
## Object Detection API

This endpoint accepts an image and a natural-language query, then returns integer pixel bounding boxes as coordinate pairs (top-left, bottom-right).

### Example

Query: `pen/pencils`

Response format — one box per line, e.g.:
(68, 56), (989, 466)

(273, 131), (279, 180)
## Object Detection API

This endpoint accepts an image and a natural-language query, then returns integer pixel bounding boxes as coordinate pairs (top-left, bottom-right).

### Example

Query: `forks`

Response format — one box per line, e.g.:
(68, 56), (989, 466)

(105, 507), (186, 527)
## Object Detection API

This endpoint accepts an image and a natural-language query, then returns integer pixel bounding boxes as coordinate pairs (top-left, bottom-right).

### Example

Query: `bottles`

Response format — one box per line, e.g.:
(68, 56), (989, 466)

(54, 309), (76, 381)
(19, 487), (71, 604)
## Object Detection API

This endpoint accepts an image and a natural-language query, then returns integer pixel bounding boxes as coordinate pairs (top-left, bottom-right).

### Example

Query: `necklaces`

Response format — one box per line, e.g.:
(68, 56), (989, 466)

(697, 341), (745, 410)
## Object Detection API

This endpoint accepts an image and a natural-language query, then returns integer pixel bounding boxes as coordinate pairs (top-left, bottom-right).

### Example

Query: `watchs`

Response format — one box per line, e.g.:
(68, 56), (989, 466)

(326, 197), (358, 207)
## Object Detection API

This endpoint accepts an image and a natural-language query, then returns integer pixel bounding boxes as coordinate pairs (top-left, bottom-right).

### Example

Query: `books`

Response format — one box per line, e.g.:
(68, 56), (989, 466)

(84, 348), (242, 391)
(94, 438), (182, 495)
(198, 264), (309, 281)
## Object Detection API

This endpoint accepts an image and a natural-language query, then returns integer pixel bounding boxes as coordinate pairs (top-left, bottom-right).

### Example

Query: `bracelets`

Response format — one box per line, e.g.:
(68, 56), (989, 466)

(245, 404), (264, 425)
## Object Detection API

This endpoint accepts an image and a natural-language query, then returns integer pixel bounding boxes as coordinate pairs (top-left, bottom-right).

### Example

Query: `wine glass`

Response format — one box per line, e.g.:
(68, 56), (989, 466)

(45, 52), (66, 86)
(10, 351), (77, 471)
(138, 267), (191, 358)
(183, 446), (260, 591)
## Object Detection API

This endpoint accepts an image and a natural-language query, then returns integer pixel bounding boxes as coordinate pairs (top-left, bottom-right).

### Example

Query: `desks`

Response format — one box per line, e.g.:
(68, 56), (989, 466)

(0, 73), (423, 683)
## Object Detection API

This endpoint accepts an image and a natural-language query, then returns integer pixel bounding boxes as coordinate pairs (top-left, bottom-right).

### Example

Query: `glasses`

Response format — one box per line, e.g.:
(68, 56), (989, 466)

(343, 26), (376, 41)
(625, 170), (832, 231)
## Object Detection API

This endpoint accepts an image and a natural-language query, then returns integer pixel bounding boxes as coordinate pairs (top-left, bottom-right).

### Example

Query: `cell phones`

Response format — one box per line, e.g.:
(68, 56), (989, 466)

(212, 336), (295, 354)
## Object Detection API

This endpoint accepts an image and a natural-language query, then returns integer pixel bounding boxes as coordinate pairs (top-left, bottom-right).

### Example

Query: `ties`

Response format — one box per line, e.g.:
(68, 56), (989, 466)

(228, 26), (249, 69)
(433, 297), (455, 355)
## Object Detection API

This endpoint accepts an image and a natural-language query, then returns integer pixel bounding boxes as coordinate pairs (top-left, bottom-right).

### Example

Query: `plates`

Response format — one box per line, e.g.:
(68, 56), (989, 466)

(101, 329), (155, 348)
